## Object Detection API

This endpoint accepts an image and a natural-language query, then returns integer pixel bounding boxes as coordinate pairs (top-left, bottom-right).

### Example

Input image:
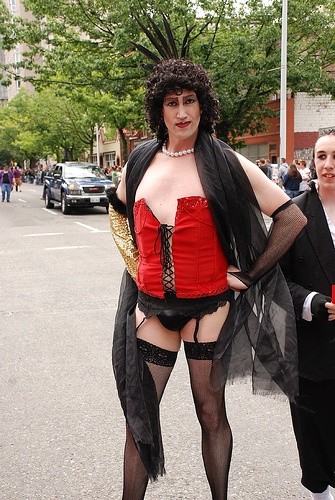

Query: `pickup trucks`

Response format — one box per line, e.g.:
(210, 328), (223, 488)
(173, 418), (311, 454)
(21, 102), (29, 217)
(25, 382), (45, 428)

(42, 161), (116, 216)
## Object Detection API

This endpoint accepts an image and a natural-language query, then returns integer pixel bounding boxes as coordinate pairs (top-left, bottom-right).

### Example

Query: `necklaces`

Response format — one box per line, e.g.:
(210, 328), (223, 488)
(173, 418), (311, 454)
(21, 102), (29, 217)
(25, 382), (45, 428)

(162, 138), (195, 157)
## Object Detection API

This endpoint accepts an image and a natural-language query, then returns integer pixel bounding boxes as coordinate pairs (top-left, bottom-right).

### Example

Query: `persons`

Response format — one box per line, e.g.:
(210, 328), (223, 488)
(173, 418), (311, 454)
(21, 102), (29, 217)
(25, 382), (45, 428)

(102, 165), (124, 187)
(8, 163), (56, 192)
(105, 58), (307, 500)
(255, 157), (314, 198)
(0, 165), (14, 202)
(266, 126), (335, 500)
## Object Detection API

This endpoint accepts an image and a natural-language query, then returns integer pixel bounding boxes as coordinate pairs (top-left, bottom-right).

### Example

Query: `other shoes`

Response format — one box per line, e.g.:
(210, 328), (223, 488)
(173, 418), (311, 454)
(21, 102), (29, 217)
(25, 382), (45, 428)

(2, 200), (4, 202)
(7, 200), (10, 202)
